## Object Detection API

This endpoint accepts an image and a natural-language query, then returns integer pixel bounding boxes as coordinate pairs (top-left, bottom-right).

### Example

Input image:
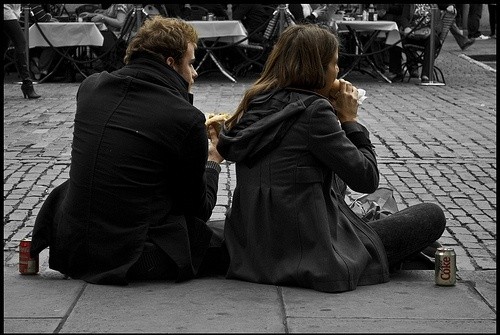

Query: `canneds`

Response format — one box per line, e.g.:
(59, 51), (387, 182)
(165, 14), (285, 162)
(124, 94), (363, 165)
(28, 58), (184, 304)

(18, 238), (39, 275)
(435, 247), (457, 286)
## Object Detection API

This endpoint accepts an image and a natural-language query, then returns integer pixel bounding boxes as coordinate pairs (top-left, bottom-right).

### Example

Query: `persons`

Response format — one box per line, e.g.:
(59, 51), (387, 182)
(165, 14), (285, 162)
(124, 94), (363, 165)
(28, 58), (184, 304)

(4, 1), (496, 98)
(28, 15), (227, 286)
(216, 23), (448, 293)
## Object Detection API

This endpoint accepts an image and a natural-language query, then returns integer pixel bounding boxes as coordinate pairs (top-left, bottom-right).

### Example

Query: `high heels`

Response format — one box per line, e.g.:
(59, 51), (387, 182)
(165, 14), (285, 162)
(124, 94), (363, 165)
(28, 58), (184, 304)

(21, 84), (41, 99)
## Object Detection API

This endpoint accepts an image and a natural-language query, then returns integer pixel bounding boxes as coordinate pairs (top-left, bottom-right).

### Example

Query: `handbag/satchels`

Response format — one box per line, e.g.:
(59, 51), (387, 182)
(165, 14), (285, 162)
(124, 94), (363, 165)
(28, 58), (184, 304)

(347, 187), (399, 224)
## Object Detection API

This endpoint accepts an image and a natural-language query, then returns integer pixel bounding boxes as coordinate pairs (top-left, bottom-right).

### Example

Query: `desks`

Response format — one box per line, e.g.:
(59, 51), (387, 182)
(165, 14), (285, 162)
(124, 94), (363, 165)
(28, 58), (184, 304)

(29, 22), (104, 83)
(191, 19), (249, 84)
(332, 19), (403, 83)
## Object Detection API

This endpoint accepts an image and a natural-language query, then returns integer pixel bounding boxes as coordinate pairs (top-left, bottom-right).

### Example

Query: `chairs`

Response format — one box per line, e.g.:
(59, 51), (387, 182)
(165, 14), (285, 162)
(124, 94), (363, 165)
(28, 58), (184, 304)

(5, 0), (456, 86)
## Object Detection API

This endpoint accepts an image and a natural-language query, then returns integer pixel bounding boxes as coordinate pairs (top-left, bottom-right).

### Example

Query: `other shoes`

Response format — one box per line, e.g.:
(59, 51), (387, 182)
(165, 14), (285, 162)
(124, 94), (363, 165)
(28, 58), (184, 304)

(475, 35), (489, 40)
(420, 78), (445, 86)
(461, 38), (475, 50)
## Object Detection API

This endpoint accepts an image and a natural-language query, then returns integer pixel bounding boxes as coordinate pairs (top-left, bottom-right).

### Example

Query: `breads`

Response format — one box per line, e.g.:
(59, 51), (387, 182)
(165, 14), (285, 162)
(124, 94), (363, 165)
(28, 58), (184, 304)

(205, 114), (232, 139)
(328, 79), (341, 99)
